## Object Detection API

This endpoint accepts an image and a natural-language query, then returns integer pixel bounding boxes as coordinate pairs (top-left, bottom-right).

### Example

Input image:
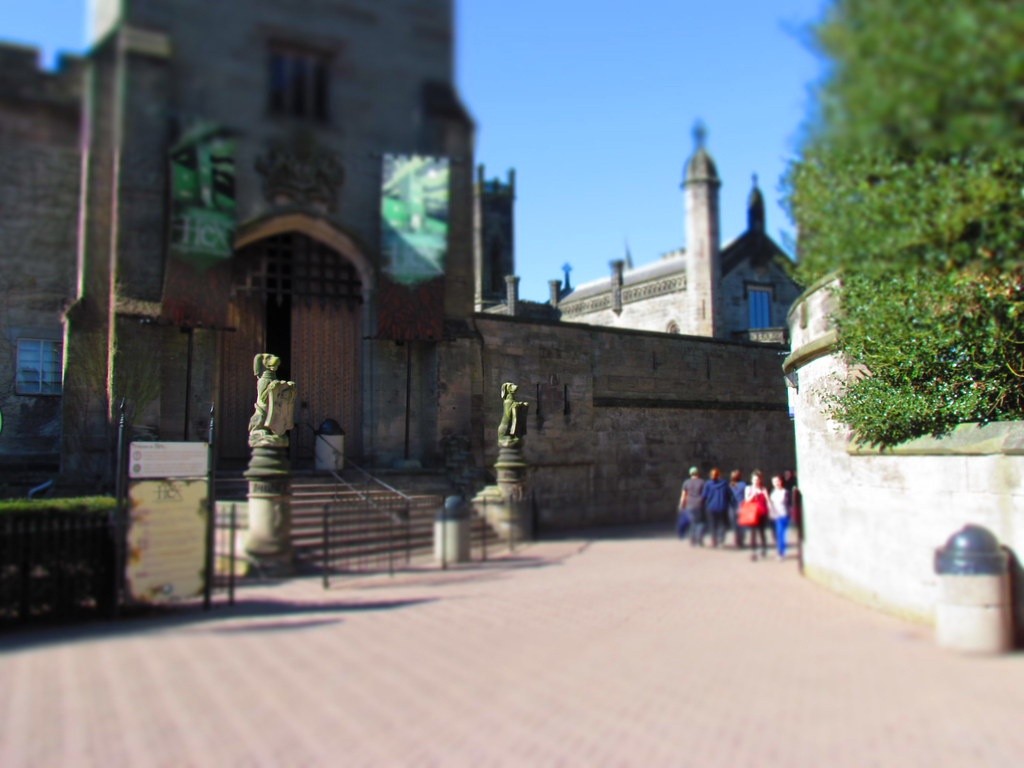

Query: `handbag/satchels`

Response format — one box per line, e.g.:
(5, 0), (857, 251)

(675, 512), (690, 539)
(737, 493), (769, 527)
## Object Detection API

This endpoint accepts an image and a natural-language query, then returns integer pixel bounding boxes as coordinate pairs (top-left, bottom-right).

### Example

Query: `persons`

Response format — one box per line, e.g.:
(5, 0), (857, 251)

(676, 466), (797, 562)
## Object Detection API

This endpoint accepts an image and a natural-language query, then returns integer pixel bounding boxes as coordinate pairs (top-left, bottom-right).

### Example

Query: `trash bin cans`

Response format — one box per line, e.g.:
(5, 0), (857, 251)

(312, 418), (346, 475)
(934, 524), (1013, 655)
(433, 495), (472, 564)
